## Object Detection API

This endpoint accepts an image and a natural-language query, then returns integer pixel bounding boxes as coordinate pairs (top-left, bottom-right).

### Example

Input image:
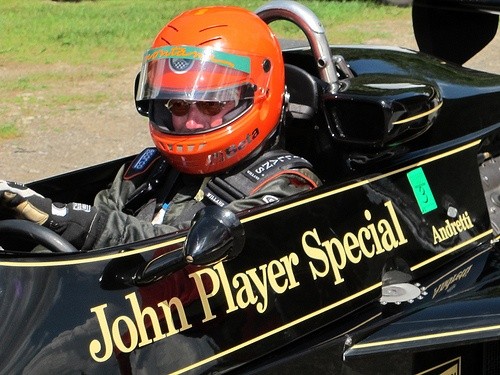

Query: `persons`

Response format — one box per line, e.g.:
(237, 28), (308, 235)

(0, 6), (326, 255)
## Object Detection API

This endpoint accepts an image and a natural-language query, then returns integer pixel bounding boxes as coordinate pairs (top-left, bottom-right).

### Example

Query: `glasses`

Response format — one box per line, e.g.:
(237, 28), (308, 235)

(160, 96), (231, 117)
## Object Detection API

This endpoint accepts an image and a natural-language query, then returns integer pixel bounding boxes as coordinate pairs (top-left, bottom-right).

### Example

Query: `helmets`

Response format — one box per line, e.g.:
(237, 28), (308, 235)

(131, 3), (284, 173)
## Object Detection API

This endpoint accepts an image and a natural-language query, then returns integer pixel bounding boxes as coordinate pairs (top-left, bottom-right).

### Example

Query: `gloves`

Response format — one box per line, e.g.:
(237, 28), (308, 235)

(0, 178), (93, 246)
